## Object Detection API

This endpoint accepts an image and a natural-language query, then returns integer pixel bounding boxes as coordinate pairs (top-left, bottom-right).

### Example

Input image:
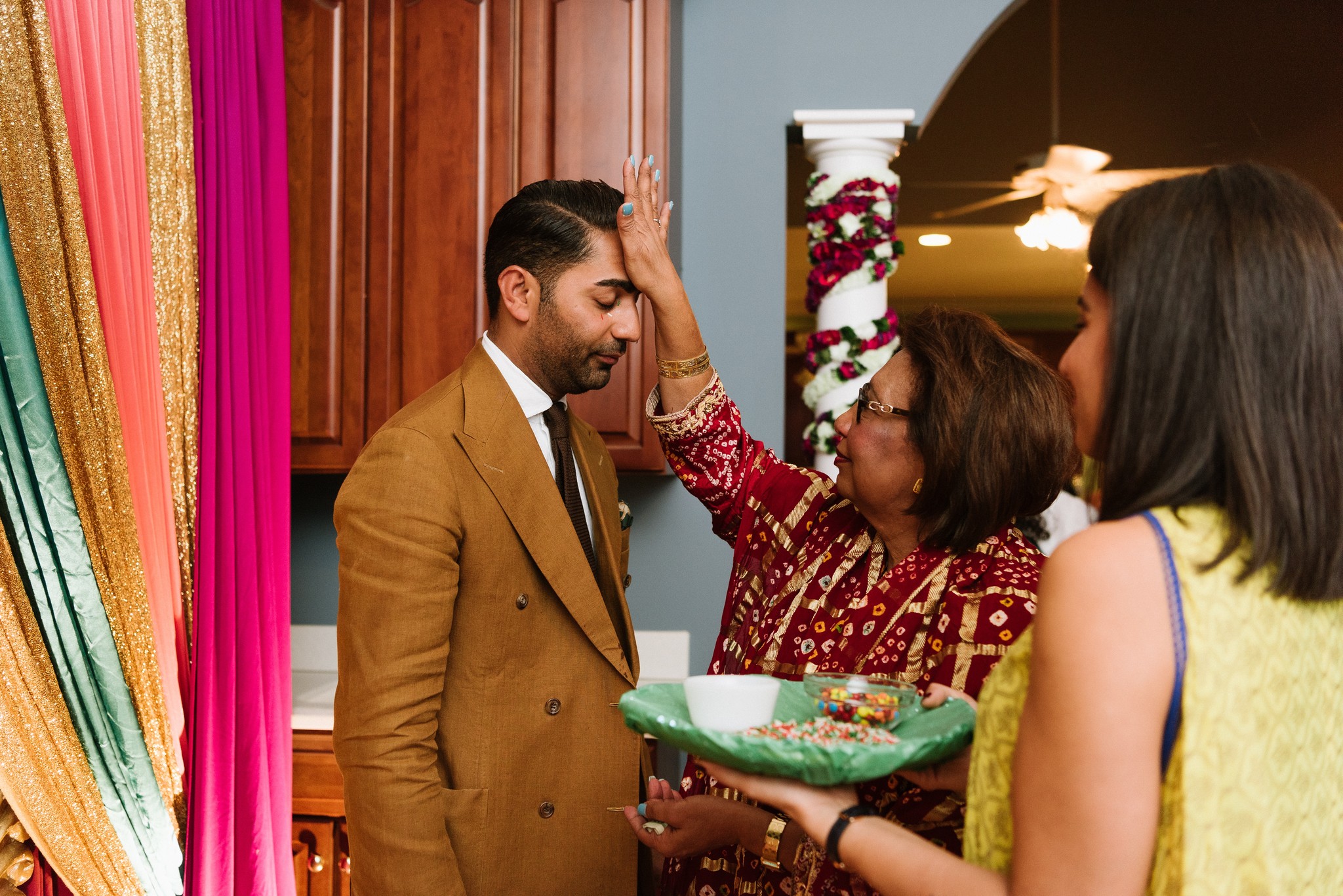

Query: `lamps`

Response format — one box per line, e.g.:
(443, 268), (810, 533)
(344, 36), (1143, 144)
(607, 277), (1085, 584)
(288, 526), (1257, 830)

(1012, 202), (1096, 253)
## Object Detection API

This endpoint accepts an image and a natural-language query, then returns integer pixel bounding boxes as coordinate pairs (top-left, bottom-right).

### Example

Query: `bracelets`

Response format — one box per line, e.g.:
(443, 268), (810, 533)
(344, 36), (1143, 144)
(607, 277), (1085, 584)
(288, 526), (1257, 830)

(656, 345), (710, 369)
(658, 355), (710, 378)
(827, 805), (878, 873)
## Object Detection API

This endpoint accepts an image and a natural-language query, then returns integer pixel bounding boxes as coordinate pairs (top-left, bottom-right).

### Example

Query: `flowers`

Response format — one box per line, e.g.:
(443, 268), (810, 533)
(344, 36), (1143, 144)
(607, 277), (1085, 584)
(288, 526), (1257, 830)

(803, 170), (904, 452)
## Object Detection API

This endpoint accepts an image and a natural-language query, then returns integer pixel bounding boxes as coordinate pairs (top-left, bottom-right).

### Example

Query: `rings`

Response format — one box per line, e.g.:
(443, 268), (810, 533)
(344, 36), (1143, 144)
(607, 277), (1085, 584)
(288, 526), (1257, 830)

(653, 218), (661, 228)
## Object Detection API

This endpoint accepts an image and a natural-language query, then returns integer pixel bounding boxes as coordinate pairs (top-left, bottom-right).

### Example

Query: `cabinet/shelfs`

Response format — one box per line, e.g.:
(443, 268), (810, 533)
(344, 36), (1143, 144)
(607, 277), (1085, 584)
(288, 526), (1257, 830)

(274, 0), (688, 475)
(290, 729), (355, 896)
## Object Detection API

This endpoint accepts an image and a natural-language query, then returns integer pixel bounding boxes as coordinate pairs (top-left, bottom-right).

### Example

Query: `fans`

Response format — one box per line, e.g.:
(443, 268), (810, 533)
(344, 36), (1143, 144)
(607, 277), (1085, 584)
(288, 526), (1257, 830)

(928, 0), (1230, 253)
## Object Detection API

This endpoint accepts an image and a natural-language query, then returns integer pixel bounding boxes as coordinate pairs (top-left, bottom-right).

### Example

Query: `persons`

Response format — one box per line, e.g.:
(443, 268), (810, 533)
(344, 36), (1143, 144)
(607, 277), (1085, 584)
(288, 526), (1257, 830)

(694, 162), (1343, 894)
(617, 153), (1088, 896)
(332, 178), (642, 896)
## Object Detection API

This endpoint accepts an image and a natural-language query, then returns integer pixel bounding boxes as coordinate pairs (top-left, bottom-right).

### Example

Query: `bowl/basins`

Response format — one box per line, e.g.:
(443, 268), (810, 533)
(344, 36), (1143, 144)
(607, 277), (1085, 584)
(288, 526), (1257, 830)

(802, 672), (917, 732)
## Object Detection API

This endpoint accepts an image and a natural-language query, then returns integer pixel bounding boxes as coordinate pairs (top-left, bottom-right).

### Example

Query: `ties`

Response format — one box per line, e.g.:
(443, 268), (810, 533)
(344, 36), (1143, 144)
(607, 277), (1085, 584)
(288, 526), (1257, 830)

(541, 402), (606, 610)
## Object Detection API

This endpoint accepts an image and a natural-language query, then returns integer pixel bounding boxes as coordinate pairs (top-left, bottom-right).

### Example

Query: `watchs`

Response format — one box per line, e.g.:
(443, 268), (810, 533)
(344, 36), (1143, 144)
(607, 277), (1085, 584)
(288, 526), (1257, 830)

(760, 813), (791, 873)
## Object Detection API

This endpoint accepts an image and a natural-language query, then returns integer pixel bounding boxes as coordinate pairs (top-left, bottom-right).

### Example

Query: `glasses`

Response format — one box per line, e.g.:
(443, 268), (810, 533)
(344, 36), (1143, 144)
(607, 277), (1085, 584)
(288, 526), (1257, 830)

(854, 383), (913, 428)
